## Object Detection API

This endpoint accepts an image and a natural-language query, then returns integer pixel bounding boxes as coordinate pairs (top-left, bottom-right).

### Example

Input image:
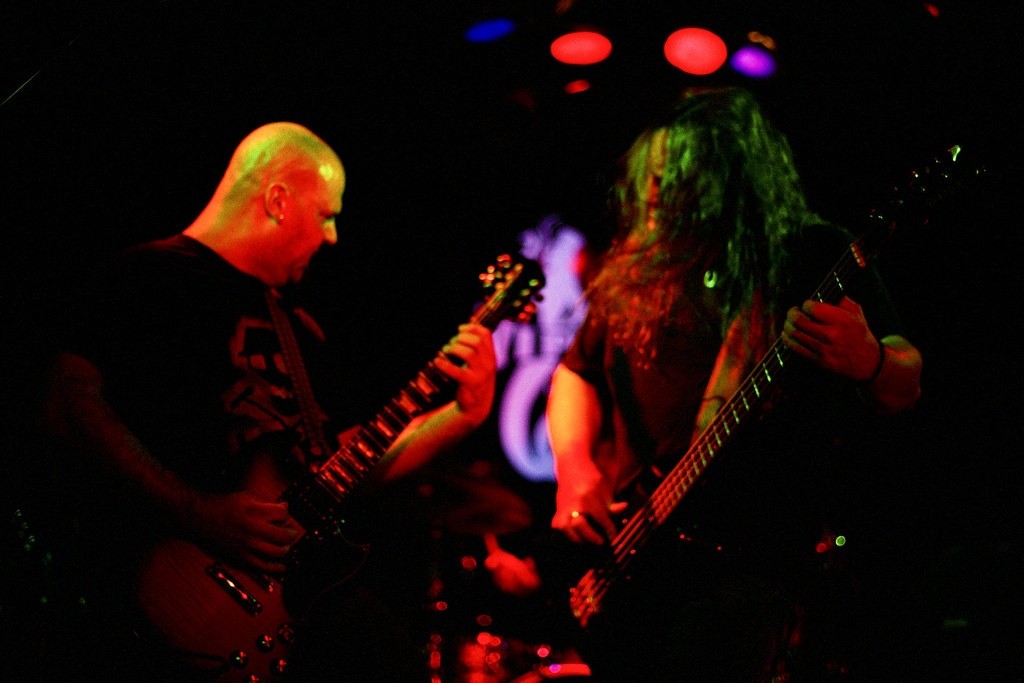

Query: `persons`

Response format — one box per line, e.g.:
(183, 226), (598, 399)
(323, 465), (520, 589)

(38, 119), (497, 683)
(544, 86), (925, 683)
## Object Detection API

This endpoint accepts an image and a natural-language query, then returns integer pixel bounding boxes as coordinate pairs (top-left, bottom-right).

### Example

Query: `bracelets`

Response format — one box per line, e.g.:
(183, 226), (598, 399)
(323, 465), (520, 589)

(859, 337), (886, 385)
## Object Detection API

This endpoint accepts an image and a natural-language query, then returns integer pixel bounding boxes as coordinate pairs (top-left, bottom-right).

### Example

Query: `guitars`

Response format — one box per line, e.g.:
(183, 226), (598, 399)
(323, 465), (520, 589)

(133, 250), (543, 683)
(567, 140), (973, 681)
